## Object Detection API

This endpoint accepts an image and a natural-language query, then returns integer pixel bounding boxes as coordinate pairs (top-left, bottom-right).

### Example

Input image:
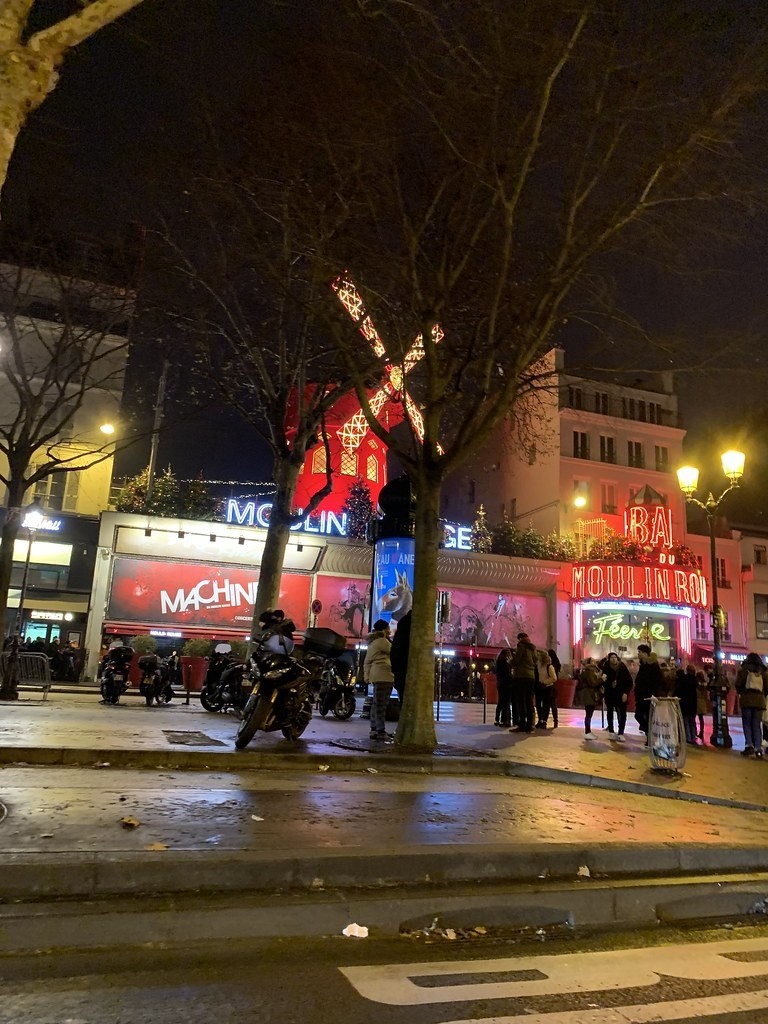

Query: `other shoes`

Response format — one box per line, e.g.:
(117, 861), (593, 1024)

(755, 751), (763, 759)
(535, 721), (547, 729)
(553, 720), (558, 728)
(494, 722), (504, 727)
(686, 738), (695, 745)
(740, 746), (754, 756)
(509, 725), (535, 734)
(698, 731), (704, 741)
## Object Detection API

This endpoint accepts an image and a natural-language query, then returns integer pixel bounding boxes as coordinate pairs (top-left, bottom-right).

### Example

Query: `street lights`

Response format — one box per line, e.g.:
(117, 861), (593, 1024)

(674, 448), (746, 750)
(0, 486), (48, 701)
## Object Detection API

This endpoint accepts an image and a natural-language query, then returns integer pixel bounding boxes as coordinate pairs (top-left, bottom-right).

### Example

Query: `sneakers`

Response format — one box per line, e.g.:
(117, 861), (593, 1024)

(608, 730), (616, 740)
(617, 735), (626, 742)
(584, 732), (600, 740)
(369, 731), (394, 744)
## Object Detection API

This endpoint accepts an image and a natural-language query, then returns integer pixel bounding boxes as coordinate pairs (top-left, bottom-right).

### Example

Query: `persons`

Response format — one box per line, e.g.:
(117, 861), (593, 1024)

(494, 647), (561, 729)
(634, 644), (669, 747)
(274, 609), (284, 623)
(734, 652), (768, 758)
(573, 657), (607, 740)
(389, 609), (412, 707)
(3, 635), (74, 680)
(505, 633), (537, 734)
(660, 662), (729, 744)
(362, 619), (394, 742)
(598, 652), (634, 743)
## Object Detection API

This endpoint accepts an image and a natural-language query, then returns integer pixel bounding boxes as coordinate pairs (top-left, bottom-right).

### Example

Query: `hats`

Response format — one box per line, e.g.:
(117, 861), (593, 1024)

(373, 619), (389, 631)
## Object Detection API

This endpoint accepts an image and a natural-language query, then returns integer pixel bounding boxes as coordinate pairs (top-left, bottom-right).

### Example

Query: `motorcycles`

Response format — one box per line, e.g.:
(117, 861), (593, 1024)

(234, 610), (347, 748)
(138, 649), (180, 706)
(200, 643), (254, 720)
(95, 640), (135, 705)
(315, 651), (361, 721)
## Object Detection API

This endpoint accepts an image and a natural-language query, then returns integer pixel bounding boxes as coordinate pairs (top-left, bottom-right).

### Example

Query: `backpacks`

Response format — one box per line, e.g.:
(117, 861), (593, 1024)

(745, 668), (763, 693)
